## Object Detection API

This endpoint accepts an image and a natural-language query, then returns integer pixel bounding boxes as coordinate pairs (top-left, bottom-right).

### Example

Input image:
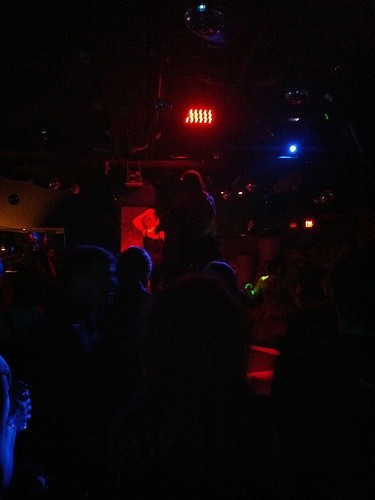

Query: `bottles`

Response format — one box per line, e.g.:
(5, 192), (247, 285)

(16, 383), (30, 432)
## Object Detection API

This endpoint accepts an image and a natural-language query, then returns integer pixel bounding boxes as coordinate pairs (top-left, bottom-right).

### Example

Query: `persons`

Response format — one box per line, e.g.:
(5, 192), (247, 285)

(0, 229), (375, 499)
(156, 168), (222, 280)
